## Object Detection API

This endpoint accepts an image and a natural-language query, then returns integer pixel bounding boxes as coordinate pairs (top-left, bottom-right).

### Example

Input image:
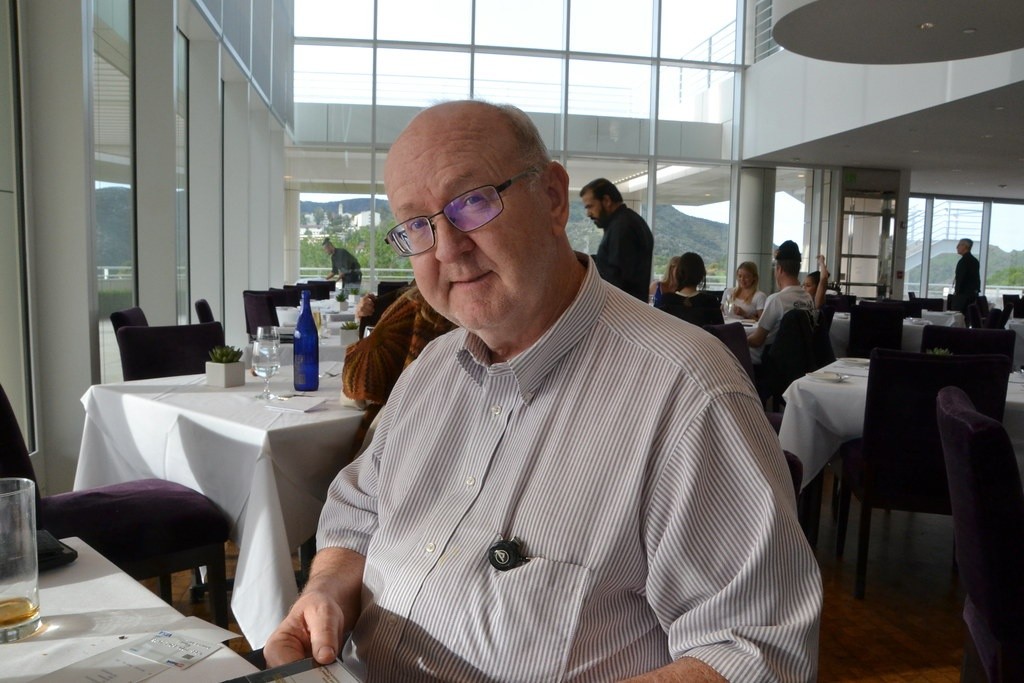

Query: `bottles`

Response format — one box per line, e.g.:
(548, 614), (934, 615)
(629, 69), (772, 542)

(293, 290), (319, 392)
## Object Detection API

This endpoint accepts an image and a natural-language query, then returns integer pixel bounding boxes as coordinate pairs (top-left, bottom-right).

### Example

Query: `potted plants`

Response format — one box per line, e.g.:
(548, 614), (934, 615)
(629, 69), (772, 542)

(335, 293), (348, 311)
(340, 322), (359, 345)
(348, 288), (359, 303)
(206, 346), (245, 388)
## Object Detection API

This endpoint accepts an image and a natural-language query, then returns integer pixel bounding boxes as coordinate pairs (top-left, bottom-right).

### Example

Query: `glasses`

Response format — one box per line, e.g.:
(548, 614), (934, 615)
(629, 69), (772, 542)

(384, 166), (538, 257)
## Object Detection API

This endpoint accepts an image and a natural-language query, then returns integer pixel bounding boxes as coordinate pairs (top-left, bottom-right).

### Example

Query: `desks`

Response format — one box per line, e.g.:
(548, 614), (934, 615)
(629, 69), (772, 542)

(70, 362), (383, 657)
(922, 309), (966, 329)
(240, 333), (362, 363)
(1002, 318), (1024, 372)
(0, 537), (259, 683)
(830, 313), (932, 355)
(777, 354), (1024, 545)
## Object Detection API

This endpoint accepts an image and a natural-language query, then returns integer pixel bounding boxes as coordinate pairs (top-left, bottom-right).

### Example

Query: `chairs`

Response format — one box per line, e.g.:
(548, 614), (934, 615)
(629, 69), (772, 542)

(0, 278), (1024, 683)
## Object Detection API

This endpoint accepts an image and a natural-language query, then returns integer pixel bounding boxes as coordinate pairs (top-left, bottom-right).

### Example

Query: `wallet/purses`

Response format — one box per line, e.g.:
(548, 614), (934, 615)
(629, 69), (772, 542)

(35, 530), (78, 571)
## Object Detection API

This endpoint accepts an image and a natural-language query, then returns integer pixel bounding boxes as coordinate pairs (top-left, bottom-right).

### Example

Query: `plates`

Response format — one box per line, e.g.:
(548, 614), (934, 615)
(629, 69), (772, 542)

(806, 373), (849, 383)
(838, 358), (870, 364)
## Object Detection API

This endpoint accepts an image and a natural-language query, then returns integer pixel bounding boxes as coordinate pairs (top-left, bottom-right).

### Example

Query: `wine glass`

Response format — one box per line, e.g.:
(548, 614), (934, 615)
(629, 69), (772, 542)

(251, 340), (281, 401)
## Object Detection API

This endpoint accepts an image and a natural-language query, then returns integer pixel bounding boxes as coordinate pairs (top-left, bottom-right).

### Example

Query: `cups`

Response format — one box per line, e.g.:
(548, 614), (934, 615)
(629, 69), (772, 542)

(257, 326), (281, 344)
(0, 477), (42, 645)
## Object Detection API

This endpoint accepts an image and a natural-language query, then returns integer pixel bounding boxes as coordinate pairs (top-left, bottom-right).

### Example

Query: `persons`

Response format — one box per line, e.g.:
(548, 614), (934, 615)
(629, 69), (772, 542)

(578, 174), (655, 303)
(322, 237), (361, 300)
(952, 237), (981, 298)
(355, 290), (374, 319)
(724, 245), (832, 367)
(261, 99), (820, 682)
(654, 251), (721, 327)
